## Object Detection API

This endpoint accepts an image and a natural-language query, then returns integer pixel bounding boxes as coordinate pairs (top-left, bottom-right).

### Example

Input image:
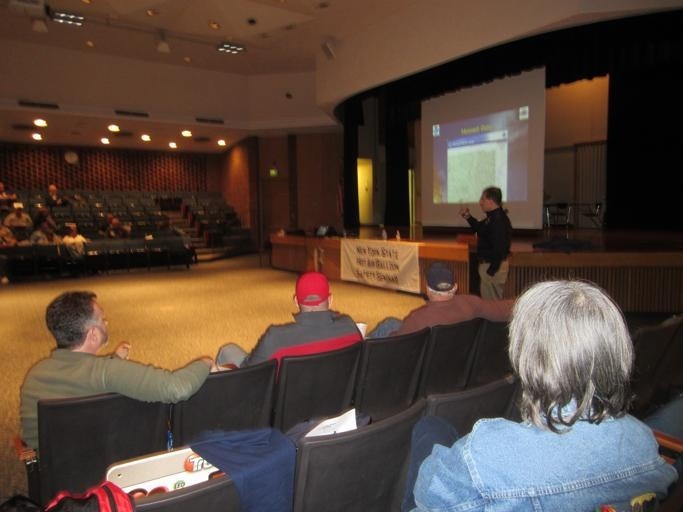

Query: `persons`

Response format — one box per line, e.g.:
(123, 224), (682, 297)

(366, 259), (457, 339)
(458, 186), (513, 302)
(413, 279), (680, 508)
(0, 181), (131, 260)
(19, 290), (216, 449)
(215, 272), (364, 368)
(158, 218), (184, 245)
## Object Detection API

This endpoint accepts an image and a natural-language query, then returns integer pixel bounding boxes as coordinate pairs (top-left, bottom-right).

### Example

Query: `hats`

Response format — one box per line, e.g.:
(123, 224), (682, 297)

(296, 271), (329, 306)
(427, 262), (455, 292)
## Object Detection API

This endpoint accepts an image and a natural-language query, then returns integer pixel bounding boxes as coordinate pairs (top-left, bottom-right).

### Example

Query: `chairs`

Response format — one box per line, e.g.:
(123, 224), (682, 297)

(0, 184), (248, 282)
(280, 318), (682, 458)
(172, 359), (280, 452)
(543, 205), (576, 241)
(18, 393), (171, 508)
(130, 441), (300, 511)
(292, 395), (428, 512)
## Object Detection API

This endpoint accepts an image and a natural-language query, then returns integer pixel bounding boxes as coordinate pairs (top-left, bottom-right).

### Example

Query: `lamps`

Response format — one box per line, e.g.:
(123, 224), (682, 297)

(155, 30), (171, 54)
(29, 19), (49, 34)
